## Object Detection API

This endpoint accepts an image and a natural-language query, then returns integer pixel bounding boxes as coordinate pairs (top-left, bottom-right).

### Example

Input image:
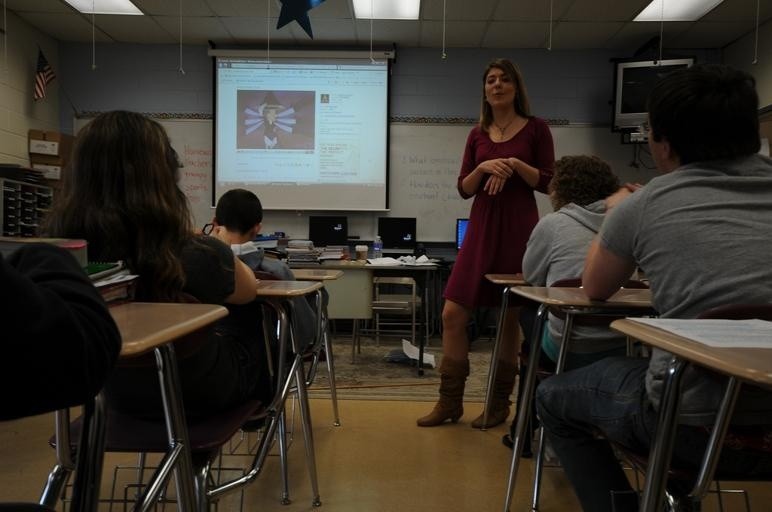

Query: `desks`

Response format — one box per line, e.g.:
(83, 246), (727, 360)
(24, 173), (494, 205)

(284, 255), (439, 359)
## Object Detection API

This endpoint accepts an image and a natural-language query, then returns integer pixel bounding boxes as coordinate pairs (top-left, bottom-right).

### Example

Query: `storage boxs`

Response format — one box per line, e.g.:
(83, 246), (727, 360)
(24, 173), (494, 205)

(27, 129), (75, 189)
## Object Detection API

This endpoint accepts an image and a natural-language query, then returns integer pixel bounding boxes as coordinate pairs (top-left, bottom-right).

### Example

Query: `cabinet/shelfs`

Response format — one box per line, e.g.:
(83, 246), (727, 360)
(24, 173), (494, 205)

(1, 180), (56, 240)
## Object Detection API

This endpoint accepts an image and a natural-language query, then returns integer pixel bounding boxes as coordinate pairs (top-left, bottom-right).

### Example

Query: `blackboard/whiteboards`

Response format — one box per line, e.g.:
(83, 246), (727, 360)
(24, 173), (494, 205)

(72, 117), (664, 247)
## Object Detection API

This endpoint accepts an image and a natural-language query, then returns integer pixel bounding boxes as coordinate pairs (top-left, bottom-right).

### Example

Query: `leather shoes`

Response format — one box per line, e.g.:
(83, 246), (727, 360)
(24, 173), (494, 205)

(502, 434), (534, 458)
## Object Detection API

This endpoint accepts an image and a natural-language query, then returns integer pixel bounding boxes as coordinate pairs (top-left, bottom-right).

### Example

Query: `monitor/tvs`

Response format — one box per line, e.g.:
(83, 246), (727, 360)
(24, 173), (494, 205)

(615, 59), (693, 128)
(457, 219), (471, 251)
(377, 217), (417, 249)
(309, 216), (348, 245)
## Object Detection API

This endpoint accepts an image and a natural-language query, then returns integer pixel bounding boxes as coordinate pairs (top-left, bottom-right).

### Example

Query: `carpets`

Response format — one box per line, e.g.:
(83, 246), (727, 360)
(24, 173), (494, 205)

(279, 330), (519, 405)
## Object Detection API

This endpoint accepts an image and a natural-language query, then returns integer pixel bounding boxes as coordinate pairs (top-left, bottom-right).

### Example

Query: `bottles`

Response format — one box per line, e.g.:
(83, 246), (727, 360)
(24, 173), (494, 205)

(372, 236), (383, 260)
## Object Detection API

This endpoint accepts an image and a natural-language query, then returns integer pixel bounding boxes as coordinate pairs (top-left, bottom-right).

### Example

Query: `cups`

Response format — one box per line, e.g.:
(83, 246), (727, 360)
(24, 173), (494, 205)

(355, 245), (369, 263)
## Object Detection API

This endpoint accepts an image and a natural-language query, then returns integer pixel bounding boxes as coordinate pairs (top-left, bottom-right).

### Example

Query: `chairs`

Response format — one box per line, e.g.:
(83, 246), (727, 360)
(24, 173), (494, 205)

(351, 277), (422, 361)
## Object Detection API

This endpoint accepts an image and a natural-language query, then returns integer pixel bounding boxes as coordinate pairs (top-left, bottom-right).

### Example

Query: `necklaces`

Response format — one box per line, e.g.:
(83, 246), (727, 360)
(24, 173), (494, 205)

(490, 117), (516, 142)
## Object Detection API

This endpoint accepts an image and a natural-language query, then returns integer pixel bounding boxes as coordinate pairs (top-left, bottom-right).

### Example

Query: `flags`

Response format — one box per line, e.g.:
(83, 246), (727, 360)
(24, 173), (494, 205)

(34, 49), (55, 103)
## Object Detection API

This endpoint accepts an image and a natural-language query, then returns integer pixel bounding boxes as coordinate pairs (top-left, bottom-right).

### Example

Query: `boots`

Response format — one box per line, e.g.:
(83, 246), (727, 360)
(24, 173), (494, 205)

(416, 352), (470, 427)
(472, 361), (518, 429)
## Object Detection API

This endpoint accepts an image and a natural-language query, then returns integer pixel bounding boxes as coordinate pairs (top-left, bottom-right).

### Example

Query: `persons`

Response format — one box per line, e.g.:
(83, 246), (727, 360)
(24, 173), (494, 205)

(536, 59), (770, 510)
(414, 58), (557, 428)
(500, 149), (652, 457)
(208, 186), (322, 412)
(37, 109), (279, 433)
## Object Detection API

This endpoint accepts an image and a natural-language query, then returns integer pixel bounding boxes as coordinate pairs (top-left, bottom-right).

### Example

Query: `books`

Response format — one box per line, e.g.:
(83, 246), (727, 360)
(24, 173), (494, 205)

(254, 232), (351, 264)
(365, 256), (403, 268)
(1, 234), (136, 310)
